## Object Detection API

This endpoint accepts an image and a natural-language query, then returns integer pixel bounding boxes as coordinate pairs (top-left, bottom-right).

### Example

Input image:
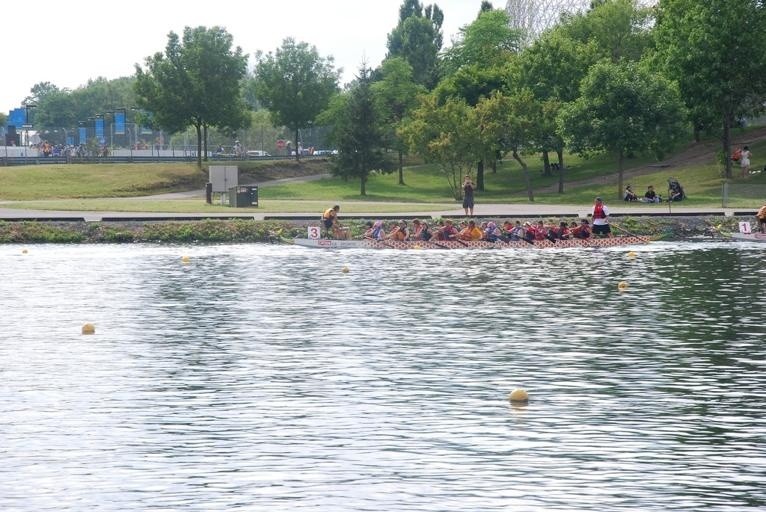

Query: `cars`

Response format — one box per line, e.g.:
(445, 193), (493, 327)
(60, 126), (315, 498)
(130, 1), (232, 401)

(246, 150), (271, 159)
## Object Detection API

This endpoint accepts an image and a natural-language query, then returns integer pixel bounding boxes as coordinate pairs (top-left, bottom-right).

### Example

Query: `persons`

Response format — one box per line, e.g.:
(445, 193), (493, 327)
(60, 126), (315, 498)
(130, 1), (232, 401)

(590, 196), (615, 238)
(216, 143), (225, 154)
(741, 145), (753, 181)
(298, 141), (302, 157)
(320, 204), (350, 240)
(286, 142), (293, 157)
(233, 139), (245, 157)
(665, 179), (685, 203)
(624, 185), (639, 202)
(32, 137), (108, 158)
(352, 218), (595, 242)
(755, 206), (766, 236)
(641, 185), (658, 204)
(460, 174), (476, 218)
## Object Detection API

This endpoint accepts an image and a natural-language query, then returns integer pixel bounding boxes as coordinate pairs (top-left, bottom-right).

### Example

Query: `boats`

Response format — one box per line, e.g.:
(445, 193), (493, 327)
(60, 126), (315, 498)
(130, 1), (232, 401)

(704, 224), (766, 240)
(268, 228), (653, 249)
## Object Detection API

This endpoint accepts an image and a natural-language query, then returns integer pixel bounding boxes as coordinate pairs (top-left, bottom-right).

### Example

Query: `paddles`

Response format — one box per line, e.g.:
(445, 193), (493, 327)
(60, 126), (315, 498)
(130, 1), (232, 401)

(509, 231), (534, 244)
(416, 235), (450, 251)
(543, 233), (557, 242)
(365, 235), (394, 249)
(453, 235), (469, 248)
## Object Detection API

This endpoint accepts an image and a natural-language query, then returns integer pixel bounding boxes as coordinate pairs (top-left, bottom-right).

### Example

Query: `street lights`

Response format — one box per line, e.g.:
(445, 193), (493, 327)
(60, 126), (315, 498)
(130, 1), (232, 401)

(71, 107), (141, 146)
(26, 105), (37, 146)
